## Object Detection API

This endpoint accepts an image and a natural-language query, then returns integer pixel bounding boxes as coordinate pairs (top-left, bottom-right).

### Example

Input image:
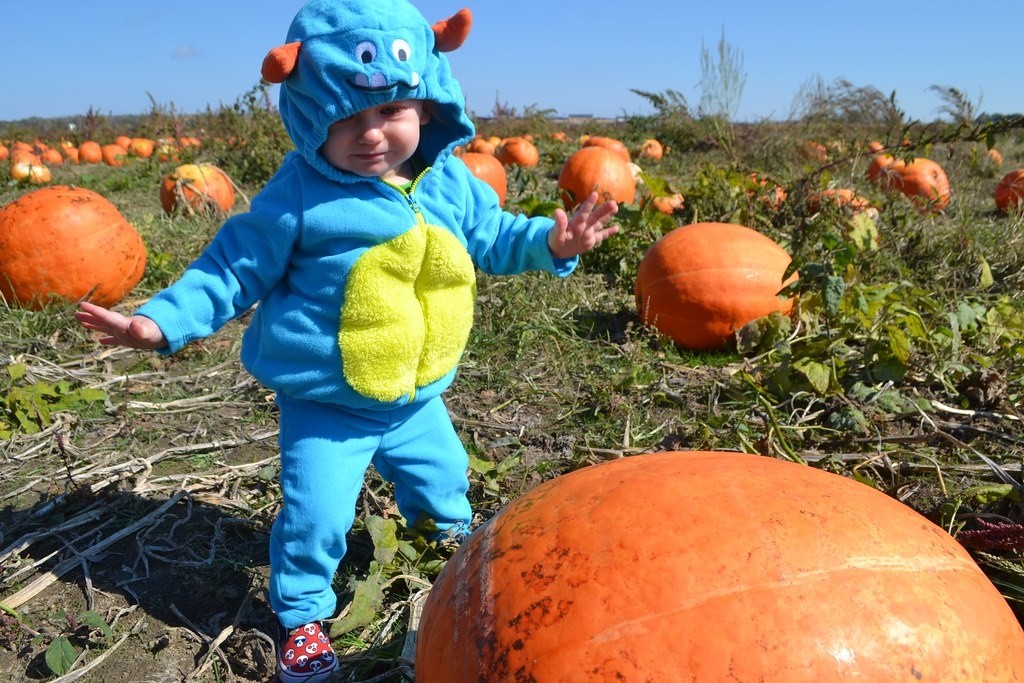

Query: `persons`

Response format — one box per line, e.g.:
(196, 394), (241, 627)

(74, 0), (620, 683)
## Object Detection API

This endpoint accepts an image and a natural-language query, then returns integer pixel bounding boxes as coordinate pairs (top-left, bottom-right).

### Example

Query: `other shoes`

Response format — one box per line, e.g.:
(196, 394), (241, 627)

(274, 623), (340, 683)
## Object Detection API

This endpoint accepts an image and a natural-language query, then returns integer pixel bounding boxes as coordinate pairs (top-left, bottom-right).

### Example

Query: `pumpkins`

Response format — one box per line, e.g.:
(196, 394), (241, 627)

(415, 452), (1024, 683)
(0, 128), (1023, 354)
